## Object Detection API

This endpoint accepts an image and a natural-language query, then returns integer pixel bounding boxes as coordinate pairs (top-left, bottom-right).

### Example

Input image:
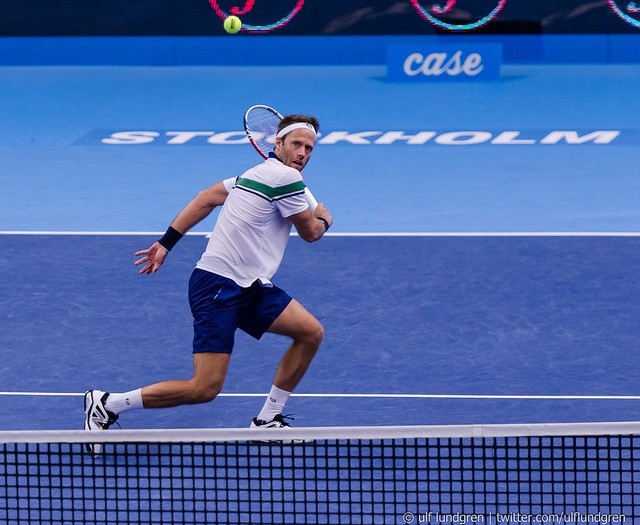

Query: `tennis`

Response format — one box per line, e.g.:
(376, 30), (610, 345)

(223, 15), (241, 34)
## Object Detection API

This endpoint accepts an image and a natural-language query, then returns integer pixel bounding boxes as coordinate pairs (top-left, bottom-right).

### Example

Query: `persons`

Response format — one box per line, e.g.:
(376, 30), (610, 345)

(83, 113), (334, 460)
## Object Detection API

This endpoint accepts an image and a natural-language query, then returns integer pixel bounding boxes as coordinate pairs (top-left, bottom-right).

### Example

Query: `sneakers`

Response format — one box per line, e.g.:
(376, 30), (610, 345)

(84, 389), (122, 458)
(249, 413), (315, 443)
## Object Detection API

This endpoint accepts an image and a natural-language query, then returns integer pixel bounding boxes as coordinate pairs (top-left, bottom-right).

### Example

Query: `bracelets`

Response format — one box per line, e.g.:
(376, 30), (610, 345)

(316, 216), (329, 233)
